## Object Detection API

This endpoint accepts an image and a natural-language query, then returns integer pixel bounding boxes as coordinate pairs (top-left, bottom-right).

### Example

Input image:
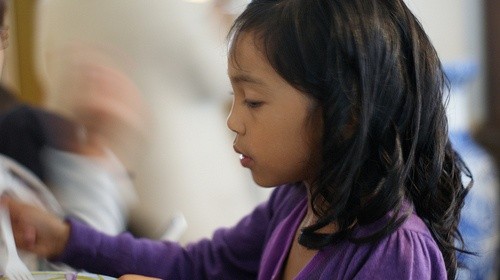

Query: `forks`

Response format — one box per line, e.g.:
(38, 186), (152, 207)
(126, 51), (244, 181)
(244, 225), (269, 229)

(0, 201), (36, 280)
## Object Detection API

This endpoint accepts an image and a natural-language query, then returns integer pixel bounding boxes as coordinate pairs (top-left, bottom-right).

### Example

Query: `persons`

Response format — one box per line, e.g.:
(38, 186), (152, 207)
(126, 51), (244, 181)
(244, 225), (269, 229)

(0, 0), (482, 280)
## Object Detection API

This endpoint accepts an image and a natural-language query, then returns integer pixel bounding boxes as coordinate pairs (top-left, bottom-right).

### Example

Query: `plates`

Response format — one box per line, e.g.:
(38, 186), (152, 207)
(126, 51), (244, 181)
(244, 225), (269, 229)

(0, 271), (120, 280)
